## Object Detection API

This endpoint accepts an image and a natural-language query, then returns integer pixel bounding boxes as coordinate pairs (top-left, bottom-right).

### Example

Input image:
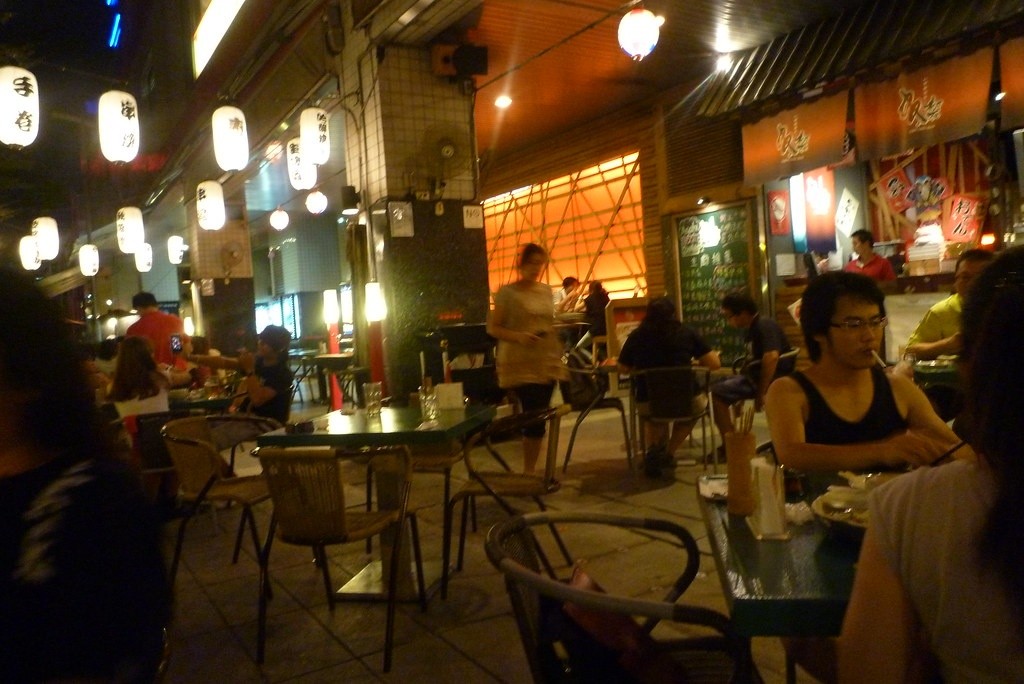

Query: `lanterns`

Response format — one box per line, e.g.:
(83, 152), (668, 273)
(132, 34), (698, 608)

(0, 65), (39, 152)
(168, 235), (183, 265)
(79, 244), (99, 277)
(32, 216), (60, 260)
(20, 236), (41, 270)
(135, 242), (152, 272)
(117, 207), (145, 254)
(99, 89), (140, 165)
(300, 108), (330, 168)
(287, 136), (317, 191)
(212, 106), (249, 171)
(196, 180), (225, 231)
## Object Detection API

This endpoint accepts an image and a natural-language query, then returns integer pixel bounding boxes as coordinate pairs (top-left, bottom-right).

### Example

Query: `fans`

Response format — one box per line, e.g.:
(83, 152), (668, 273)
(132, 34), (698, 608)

(219, 240), (243, 285)
(415, 119), (475, 201)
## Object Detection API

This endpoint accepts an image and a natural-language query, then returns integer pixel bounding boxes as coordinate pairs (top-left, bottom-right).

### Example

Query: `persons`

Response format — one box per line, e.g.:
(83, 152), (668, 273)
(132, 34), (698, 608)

(904, 248), (996, 360)
(844, 229), (896, 280)
(834, 245), (1024, 684)
(486, 243), (562, 500)
(75, 291), (294, 477)
(695, 291), (796, 464)
(617, 295), (720, 478)
(0, 263), (170, 684)
(766, 271), (978, 684)
(560, 277), (609, 403)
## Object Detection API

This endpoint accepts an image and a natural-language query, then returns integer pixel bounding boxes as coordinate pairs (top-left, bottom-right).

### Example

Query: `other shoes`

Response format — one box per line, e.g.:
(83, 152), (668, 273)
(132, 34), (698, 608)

(644, 446), (675, 478)
(709, 446), (726, 462)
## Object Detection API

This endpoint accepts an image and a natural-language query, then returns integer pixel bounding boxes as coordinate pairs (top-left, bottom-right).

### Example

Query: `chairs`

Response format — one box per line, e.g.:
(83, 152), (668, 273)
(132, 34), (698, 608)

(92, 318), (963, 682)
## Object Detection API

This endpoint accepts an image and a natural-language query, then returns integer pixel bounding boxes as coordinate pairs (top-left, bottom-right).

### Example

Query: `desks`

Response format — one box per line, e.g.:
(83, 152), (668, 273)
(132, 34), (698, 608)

(287, 345), (319, 399)
(698, 462), (909, 640)
(257, 401), (493, 604)
(170, 394), (234, 416)
(911, 363), (961, 423)
(597, 354), (728, 448)
(312, 352), (355, 401)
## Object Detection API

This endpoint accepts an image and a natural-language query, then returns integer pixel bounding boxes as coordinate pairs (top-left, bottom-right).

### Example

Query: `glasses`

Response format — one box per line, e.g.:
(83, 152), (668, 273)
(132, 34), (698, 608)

(829, 316), (888, 335)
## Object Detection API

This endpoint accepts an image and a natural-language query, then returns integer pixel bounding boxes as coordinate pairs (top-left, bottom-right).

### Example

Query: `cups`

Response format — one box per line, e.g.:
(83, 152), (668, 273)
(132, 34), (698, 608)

(724, 432), (756, 515)
(207, 376), (220, 400)
(362, 382), (382, 416)
(418, 385), (440, 420)
(746, 462), (796, 541)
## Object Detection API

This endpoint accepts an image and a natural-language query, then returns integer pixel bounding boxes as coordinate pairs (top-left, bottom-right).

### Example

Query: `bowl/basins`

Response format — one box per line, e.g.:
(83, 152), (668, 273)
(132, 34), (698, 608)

(812, 490), (866, 537)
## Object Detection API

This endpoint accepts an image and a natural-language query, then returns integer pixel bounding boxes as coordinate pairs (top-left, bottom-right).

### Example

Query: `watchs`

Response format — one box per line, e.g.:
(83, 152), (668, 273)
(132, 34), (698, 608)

(248, 372), (255, 376)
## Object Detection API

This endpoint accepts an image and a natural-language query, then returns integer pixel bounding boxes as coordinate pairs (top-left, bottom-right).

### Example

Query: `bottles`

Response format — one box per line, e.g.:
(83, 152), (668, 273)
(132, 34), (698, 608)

(904, 348), (916, 364)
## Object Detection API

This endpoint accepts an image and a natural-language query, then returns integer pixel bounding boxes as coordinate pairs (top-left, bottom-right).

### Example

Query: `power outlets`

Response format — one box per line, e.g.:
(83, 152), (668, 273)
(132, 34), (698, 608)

(415, 191), (429, 200)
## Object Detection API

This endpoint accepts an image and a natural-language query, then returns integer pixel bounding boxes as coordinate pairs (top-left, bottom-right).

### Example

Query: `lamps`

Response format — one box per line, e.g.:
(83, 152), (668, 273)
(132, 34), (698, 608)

(696, 195), (710, 207)
(341, 186), (360, 216)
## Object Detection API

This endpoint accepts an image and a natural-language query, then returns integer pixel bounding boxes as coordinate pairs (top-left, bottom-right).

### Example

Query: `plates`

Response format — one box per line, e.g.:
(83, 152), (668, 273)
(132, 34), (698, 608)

(555, 312), (586, 323)
(849, 474), (904, 490)
